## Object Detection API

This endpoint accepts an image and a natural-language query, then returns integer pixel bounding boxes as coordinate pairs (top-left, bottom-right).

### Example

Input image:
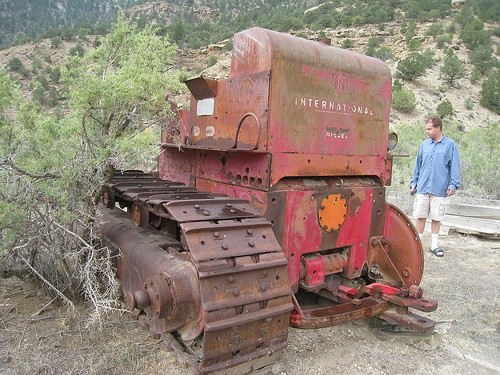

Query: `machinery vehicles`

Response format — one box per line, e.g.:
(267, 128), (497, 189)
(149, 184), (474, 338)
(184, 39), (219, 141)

(84, 27), (438, 374)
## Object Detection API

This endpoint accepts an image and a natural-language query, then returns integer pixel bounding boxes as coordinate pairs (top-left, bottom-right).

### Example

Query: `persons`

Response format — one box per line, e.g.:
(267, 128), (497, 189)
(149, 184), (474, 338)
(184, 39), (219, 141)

(410, 115), (461, 256)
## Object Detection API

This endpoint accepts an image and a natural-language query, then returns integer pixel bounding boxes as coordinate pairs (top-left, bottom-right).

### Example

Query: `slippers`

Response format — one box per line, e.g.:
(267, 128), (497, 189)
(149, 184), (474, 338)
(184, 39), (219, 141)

(429, 247), (444, 257)
